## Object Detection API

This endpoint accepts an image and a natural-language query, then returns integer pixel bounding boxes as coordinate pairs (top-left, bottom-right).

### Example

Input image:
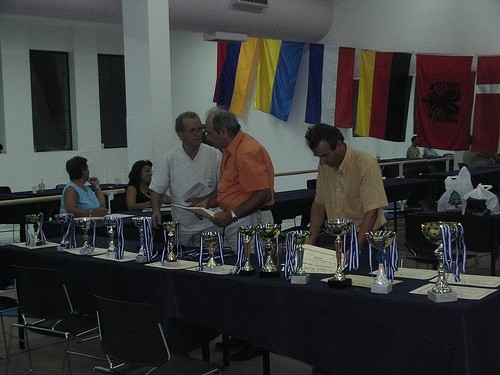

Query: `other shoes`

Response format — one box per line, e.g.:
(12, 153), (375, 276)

(214, 337), (243, 350)
(230, 343), (264, 362)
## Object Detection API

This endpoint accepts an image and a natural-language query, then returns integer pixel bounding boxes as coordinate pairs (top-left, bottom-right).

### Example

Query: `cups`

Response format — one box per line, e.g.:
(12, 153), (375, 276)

(32, 186), (37, 194)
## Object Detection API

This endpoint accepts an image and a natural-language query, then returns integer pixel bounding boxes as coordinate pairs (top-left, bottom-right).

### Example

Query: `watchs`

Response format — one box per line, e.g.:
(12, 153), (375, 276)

(230, 210), (238, 222)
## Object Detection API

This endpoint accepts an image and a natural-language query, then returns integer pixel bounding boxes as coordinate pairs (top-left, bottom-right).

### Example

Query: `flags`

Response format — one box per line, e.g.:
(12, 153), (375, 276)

(356, 49), (413, 143)
(415, 53), (475, 152)
(212, 38), (260, 117)
(253, 38), (305, 122)
(305, 44), (357, 129)
(471, 56), (500, 154)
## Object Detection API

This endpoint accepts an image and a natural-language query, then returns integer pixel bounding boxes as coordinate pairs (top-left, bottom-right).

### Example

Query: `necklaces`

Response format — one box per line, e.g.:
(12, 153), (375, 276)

(138, 185), (152, 198)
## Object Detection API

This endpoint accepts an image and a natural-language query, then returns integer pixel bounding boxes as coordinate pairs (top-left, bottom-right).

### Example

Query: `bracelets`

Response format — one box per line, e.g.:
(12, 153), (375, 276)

(89, 209), (94, 217)
(93, 184), (101, 189)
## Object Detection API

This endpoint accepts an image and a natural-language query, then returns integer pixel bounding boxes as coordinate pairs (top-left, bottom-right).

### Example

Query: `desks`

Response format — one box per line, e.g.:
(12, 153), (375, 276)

(0, 235), (500, 375)
(383, 178), (438, 230)
(35, 189), (316, 236)
(376, 156), (454, 179)
(405, 212), (499, 276)
(423, 166), (499, 188)
(1, 183), (125, 223)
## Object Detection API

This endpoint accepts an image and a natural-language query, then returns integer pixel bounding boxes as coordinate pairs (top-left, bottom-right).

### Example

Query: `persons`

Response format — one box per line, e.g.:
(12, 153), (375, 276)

(204, 106), (279, 362)
(304, 124), (387, 265)
(59, 156), (108, 217)
(149, 112), (224, 247)
(406, 136), (439, 159)
(120, 160), (171, 212)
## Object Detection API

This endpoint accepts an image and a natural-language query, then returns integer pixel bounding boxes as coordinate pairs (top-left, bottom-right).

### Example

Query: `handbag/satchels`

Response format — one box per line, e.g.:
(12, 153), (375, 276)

(438, 166), (473, 212)
(461, 183), (500, 217)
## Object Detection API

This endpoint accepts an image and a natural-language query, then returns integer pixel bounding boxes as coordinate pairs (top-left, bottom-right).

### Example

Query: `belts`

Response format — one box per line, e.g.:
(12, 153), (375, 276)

(260, 205), (271, 212)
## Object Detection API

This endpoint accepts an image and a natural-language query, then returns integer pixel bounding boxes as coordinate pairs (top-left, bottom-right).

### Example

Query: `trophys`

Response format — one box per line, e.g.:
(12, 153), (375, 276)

(319, 219), (355, 288)
(132, 216), (157, 263)
(160, 221), (183, 267)
(290, 230), (312, 284)
(76, 217), (96, 253)
(200, 231), (222, 268)
(104, 216), (125, 259)
(364, 229), (395, 294)
(57, 213), (76, 250)
(254, 223), (280, 278)
(25, 214), (46, 247)
(421, 222), (460, 303)
(236, 224), (256, 275)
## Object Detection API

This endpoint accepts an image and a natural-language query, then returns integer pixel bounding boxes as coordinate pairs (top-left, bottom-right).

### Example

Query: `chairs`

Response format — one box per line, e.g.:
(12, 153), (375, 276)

(0, 179), (500, 375)
